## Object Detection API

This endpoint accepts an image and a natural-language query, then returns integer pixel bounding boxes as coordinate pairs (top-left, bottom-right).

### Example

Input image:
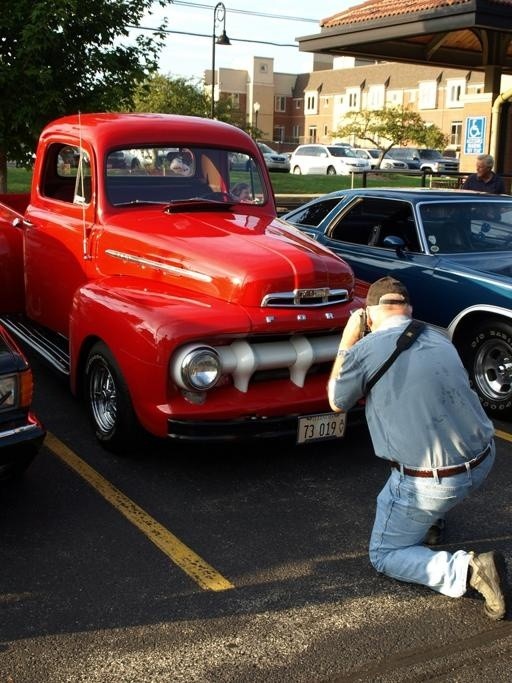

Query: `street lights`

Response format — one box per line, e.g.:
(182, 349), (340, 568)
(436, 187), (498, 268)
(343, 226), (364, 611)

(211, 2), (232, 117)
(253, 102), (260, 129)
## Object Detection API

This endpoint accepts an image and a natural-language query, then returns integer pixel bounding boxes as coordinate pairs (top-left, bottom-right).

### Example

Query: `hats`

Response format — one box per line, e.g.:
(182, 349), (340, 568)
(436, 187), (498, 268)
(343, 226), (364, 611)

(365, 275), (412, 308)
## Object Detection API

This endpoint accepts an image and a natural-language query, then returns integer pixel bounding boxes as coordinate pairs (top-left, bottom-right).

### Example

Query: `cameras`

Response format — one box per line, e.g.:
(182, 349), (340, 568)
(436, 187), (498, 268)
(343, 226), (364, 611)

(350, 307), (371, 332)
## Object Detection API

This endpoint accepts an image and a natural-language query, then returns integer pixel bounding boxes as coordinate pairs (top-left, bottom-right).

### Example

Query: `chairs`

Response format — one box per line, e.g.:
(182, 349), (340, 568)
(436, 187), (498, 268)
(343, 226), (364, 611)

(442, 222), (474, 248)
(368, 222), (416, 252)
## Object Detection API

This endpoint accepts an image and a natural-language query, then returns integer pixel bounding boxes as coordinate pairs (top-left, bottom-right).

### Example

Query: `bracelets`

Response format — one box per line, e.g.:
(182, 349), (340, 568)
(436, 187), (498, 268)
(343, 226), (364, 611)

(336, 349), (347, 358)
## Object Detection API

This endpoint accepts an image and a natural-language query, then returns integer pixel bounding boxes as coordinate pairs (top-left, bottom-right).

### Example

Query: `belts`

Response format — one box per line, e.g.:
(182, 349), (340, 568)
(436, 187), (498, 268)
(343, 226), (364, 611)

(393, 442), (494, 479)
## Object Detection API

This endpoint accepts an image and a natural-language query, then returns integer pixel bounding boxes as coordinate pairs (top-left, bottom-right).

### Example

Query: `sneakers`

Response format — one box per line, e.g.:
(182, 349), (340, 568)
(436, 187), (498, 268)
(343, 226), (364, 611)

(466, 549), (510, 620)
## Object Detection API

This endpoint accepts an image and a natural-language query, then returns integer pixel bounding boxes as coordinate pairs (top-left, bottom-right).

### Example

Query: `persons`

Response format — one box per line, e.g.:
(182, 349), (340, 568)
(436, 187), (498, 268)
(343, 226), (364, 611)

(463, 154), (506, 193)
(228, 182), (262, 204)
(325, 277), (511, 623)
